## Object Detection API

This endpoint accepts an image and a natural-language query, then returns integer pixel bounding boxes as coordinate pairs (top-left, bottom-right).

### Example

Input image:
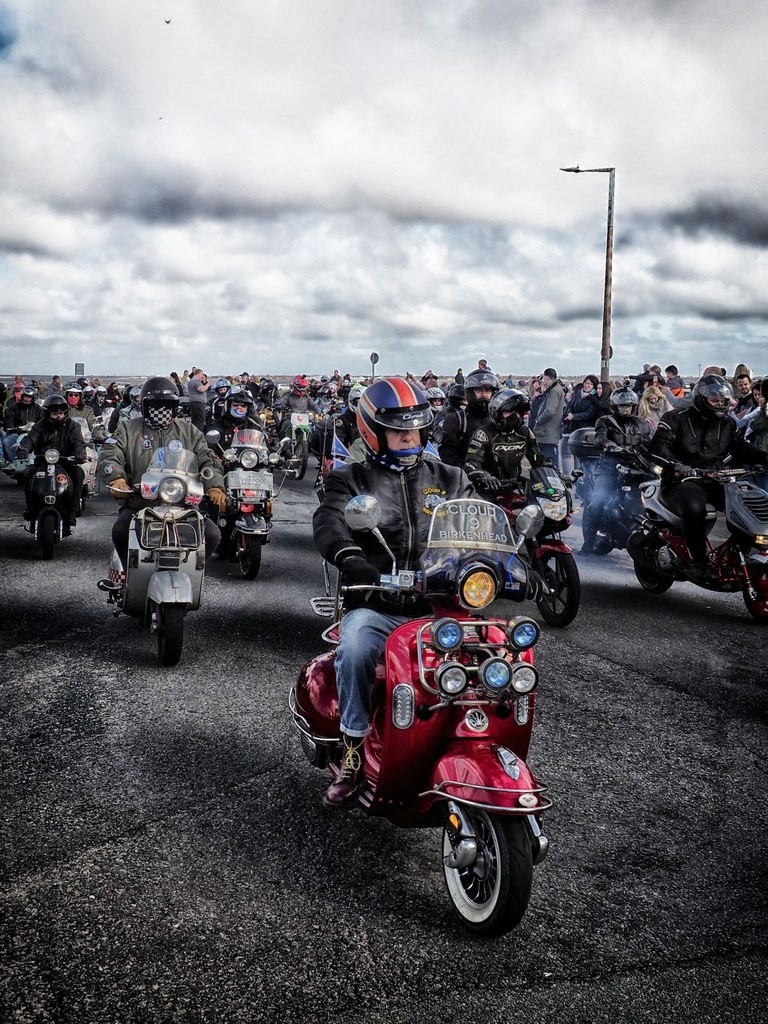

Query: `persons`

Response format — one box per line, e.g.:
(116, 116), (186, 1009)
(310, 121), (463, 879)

(312, 376), (552, 809)
(406, 359), (501, 467)
(502, 364), (733, 557)
(97, 376), (226, 573)
(648, 374), (768, 580)
(170, 367), (380, 542)
(463, 389), (572, 504)
(726, 364), (768, 492)
(0, 374), (142, 526)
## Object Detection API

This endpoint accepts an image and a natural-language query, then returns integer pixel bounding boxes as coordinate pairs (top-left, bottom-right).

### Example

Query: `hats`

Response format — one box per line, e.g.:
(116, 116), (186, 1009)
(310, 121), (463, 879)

(239, 372), (250, 377)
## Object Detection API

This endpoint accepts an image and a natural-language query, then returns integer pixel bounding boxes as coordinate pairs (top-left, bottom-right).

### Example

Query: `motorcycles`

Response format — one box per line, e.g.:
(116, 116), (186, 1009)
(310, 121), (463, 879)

(0, 370), (768, 668)
(287, 492), (552, 942)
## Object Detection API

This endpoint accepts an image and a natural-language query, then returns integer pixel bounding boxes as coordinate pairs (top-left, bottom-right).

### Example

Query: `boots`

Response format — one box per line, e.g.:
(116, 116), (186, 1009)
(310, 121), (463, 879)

(323, 735), (369, 810)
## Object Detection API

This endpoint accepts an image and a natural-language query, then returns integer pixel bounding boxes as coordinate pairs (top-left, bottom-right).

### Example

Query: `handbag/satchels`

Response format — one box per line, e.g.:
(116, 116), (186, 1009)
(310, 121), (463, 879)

(750, 401), (768, 432)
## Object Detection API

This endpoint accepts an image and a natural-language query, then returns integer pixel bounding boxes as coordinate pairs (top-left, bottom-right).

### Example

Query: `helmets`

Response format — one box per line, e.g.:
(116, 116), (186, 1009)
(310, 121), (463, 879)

(12, 368), (736, 456)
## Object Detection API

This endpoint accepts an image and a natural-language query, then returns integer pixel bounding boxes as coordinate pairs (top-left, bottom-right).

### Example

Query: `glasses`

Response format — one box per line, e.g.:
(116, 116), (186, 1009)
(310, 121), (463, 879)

(47, 404), (68, 413)
(296, 384), (306, 390)
(112, 385), (117, 388)
(232, 402), (247, 407)
(67, 393), (81, 398)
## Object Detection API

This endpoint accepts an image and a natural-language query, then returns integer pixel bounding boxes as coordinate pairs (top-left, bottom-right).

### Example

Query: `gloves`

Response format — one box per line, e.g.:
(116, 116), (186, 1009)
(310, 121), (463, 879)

(559, 475), (573, 489)
(108, 478), (131, 499)
(341, 560), (380, 595)
(206, 487), (227, 507)
(481, 475), (503, 493)
(16, 450), (30, 462)
(75, 451), (88, 461)
(275, 404), (282, 412)
(526, 569), (551, 604)
(673, 462), (695, 481)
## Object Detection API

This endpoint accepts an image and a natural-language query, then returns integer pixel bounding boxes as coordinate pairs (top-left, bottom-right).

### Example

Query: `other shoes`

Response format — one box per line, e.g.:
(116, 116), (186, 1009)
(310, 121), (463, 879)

(690, 546), (709, 571)
(578, 540), (592, 555)
(1, 464), (16, 473)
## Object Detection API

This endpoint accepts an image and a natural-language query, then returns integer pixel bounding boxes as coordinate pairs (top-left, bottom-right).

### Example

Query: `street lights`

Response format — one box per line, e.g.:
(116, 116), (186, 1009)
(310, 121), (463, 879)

(560, 167), (615, 382)
